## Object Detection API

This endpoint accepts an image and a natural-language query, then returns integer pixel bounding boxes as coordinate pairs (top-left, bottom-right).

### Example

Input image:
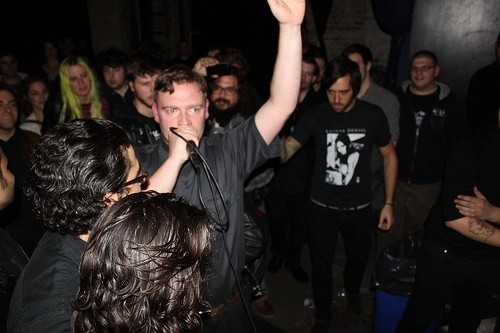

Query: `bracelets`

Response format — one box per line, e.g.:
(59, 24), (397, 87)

(385, 202), (393, 206)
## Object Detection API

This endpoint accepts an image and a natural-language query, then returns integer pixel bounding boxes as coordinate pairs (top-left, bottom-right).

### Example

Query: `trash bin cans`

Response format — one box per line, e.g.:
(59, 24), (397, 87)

(377, 234), (460, 332)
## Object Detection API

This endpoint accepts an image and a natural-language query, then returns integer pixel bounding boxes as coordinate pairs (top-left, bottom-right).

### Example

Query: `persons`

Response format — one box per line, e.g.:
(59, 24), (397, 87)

(0, 42), (500, 333)
(134, 0), (306, 333)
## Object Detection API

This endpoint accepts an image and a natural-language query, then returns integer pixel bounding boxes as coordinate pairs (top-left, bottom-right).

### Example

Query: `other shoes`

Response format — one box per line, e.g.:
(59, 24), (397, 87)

(285, 260), (309, 283)
(347, 294), (361, 315)
(267, 253), (284, 274)
(256, 300), (276, 318)
(312, 320), (328, 333)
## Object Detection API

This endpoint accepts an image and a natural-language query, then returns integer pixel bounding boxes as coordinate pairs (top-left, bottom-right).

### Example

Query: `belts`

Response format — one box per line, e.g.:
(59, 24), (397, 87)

(310, 197), (369, 212)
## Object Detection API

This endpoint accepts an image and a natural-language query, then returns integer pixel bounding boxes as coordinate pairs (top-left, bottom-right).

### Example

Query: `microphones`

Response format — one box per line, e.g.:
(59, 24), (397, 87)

(186, 140), (201, 168)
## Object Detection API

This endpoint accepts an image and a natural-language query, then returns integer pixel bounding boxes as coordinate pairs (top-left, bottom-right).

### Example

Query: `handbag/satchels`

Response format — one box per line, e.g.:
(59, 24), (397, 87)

(242, 212), (265, 265)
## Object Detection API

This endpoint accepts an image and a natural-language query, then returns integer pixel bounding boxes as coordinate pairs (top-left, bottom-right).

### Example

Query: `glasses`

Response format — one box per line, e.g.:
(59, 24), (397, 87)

(108, 166), (149, 206)
(411, 65), (436, 72)
(213, 86), (238, 95)
